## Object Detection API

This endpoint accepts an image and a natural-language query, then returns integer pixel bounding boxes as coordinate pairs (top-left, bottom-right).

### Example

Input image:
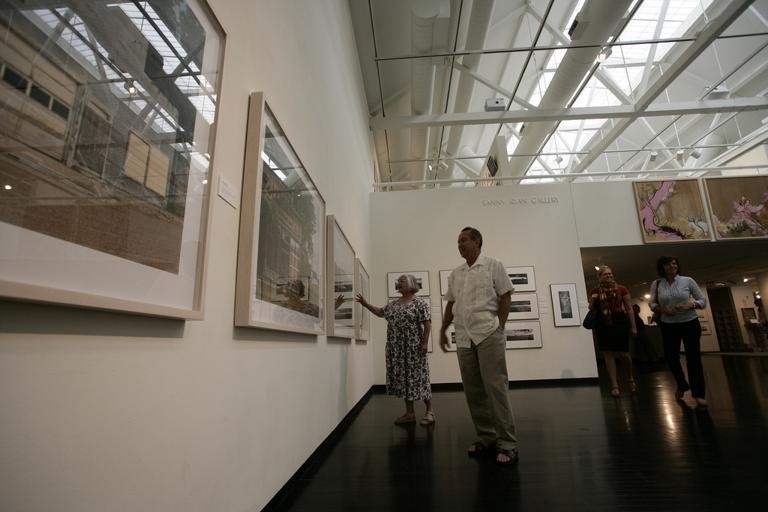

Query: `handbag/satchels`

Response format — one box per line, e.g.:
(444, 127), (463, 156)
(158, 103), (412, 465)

(650, 279), (663, 329)
(583, 290), (602, 330)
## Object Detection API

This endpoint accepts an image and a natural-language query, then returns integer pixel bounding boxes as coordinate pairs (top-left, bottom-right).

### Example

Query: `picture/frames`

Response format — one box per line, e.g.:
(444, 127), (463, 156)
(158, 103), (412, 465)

(235, 92), (328, 336)
(438, 269), (455, 297)
(502, 293), (541, 321)
(549, 283), (581, 327)
(386, 271), (430, 298)
(356, 258), (371, 342)
(326, 215), (356, 340)
(502, 264), (537, 292)
(0, 2), (230, 320)
(502, 321), (543, 351)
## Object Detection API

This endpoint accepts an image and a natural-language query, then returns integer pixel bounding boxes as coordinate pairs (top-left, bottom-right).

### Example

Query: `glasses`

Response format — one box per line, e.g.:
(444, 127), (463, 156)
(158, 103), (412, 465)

(398, 279), (407, 283)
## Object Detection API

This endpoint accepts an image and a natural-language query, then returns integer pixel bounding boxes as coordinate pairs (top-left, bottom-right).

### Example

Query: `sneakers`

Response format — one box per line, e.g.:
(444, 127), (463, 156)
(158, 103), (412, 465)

(695, 397), (707, 405)
(675, 387), (688, 401)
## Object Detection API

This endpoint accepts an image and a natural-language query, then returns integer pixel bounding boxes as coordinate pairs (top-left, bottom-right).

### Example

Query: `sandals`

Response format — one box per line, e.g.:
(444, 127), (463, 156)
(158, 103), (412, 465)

(496, 447), (518, 466)
(628, 381), (640, 395)
(421, 411), (435, 425)
(395, 413), (417, 424)
(610, 386), (621, 398)
(467, 441), (495, 456)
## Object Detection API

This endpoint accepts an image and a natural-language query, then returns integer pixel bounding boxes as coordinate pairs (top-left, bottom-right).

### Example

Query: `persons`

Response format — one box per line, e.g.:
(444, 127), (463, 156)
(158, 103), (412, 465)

(439, 226), (519, 465)
(633, 304), (653, 363)
(355, 274), (436, 425)
(282, 279), (348, 317)
(588, 265), (637, 397)
(753, 292), (767, 326)
(648, 302), (661, 327)
(648, 256), (707, 408)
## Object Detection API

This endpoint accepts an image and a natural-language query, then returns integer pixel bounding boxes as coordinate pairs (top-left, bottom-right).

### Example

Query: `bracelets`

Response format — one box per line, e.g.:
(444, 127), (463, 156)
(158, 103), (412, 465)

(690, 299), (700, 311)
